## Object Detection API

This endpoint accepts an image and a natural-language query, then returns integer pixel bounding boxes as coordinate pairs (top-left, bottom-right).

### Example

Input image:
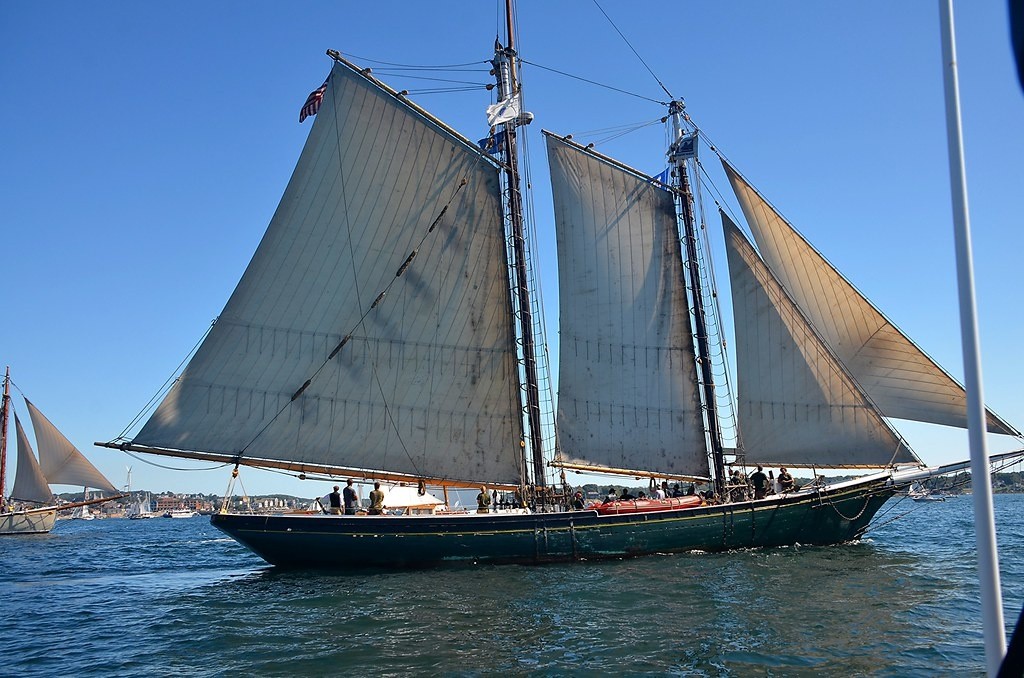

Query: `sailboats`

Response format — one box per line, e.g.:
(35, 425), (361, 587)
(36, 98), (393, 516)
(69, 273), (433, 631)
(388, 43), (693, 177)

(91, 0), (1024, 574)
(282, 478), (471, 515)
(908, 479), (946, 503)
(0, 364), (132, 534)
(69, 486), (96, 520)
(126, 497), (156, 519)
(96, 491), (107, 520)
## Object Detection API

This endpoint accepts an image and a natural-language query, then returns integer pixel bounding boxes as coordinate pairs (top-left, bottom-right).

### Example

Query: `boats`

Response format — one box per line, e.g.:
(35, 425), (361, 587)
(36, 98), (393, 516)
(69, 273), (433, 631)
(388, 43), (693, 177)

(584, 495), (706, 514)
(163, 509), (198, 519)
(946, 496), (959, 498)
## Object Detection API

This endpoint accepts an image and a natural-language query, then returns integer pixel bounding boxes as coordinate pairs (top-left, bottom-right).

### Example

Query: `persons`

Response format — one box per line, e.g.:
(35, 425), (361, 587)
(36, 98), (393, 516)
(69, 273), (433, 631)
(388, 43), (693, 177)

(620, 489), (638, 501)
(343, 478), (358, 515)
(477, 485), (492, 513)
(637, 483), (695, 500)
(733, 465), (794, 499)
(574, 491), (584, 509)
(369, 482), (384, 515)
(329, 485), (342, 515)
(602, 488), (617, 505)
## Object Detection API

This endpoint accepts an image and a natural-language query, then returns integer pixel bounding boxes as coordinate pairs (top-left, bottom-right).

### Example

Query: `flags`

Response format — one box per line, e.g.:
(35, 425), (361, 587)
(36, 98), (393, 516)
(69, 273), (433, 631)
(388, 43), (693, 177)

(487, 93), (519, 125)
(478, 130), (504, 154)
(300, 70), (331, 123)
